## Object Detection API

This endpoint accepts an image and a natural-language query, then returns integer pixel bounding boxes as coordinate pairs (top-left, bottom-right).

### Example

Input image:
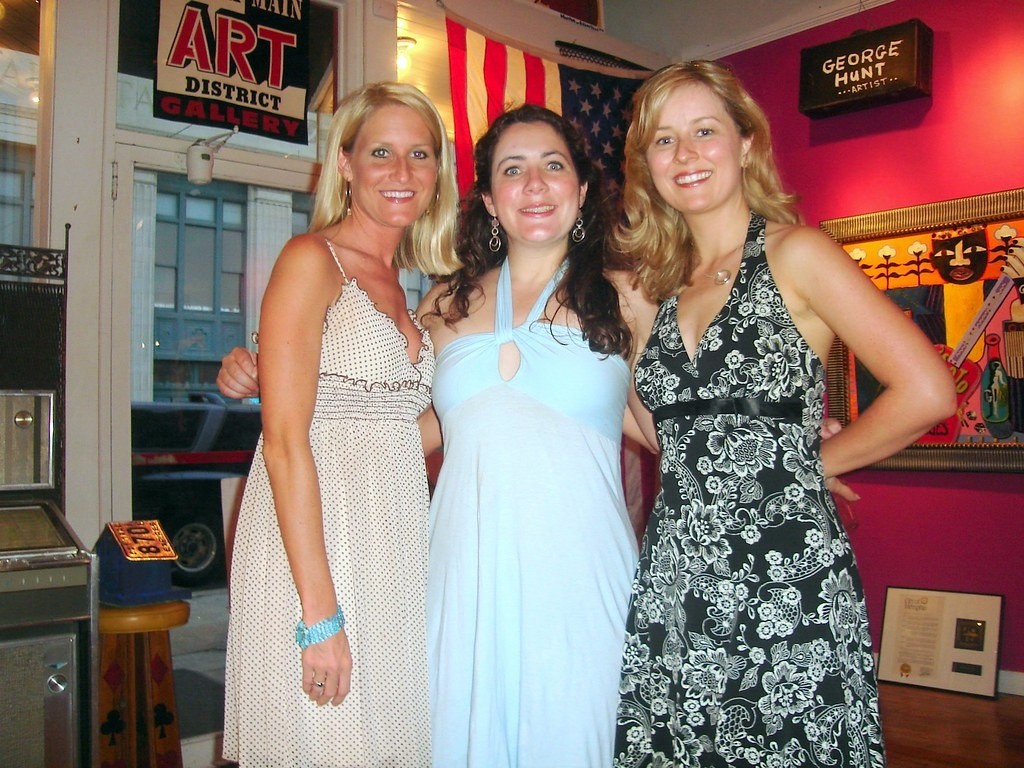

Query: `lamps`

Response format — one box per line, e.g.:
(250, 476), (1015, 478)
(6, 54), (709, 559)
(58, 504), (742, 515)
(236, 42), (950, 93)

(187, 128), (237, 184)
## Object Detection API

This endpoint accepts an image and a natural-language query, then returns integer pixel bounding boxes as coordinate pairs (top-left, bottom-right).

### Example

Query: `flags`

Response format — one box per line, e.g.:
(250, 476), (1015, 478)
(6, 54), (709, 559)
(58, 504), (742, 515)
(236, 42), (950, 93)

(446, 11), (647, 240)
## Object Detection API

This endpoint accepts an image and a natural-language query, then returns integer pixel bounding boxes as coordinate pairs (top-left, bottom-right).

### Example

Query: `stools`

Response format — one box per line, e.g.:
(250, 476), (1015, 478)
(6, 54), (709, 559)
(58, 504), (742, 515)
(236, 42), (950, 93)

(93, 600), (191, 768)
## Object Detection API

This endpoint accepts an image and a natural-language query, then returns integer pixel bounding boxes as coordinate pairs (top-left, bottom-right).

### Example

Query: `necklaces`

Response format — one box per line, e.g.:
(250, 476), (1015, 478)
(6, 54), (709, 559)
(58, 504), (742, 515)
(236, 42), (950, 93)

(696, 233), (746, 285)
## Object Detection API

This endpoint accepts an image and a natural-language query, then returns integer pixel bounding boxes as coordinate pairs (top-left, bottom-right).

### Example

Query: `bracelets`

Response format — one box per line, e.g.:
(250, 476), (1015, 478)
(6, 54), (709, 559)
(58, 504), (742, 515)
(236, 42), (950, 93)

(295, 605), (345, 649)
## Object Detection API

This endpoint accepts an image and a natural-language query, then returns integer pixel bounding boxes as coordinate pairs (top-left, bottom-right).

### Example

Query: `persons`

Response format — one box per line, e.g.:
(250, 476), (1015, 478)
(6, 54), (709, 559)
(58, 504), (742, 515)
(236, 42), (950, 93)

(222, 82), (464, 768)
(607, 60), (957, 768)
(216, 104), (842, 768)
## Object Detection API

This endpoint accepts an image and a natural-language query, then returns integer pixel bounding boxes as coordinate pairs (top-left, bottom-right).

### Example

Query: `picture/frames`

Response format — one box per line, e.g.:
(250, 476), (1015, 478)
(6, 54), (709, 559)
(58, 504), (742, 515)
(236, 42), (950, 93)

(818, 185), (1024, 477)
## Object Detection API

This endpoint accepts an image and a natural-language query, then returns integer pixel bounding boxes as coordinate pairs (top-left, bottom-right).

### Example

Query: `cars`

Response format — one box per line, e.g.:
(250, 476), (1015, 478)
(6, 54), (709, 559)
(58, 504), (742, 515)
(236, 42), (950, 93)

(130, 390), (268, 589)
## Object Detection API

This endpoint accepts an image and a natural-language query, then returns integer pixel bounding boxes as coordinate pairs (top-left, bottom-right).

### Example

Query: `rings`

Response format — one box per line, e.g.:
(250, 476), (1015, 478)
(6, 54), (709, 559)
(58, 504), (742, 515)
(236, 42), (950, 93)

(313, 679), (325, 687)
